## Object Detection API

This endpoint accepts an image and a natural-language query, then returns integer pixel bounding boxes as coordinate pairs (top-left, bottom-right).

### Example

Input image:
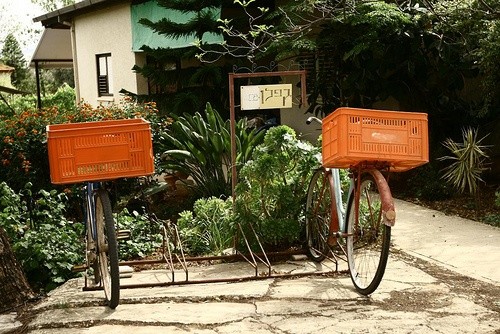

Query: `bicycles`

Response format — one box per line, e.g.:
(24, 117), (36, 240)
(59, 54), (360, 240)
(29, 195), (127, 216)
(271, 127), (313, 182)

(301, 116), (395, 295)
(41, 135), (132, 310)
